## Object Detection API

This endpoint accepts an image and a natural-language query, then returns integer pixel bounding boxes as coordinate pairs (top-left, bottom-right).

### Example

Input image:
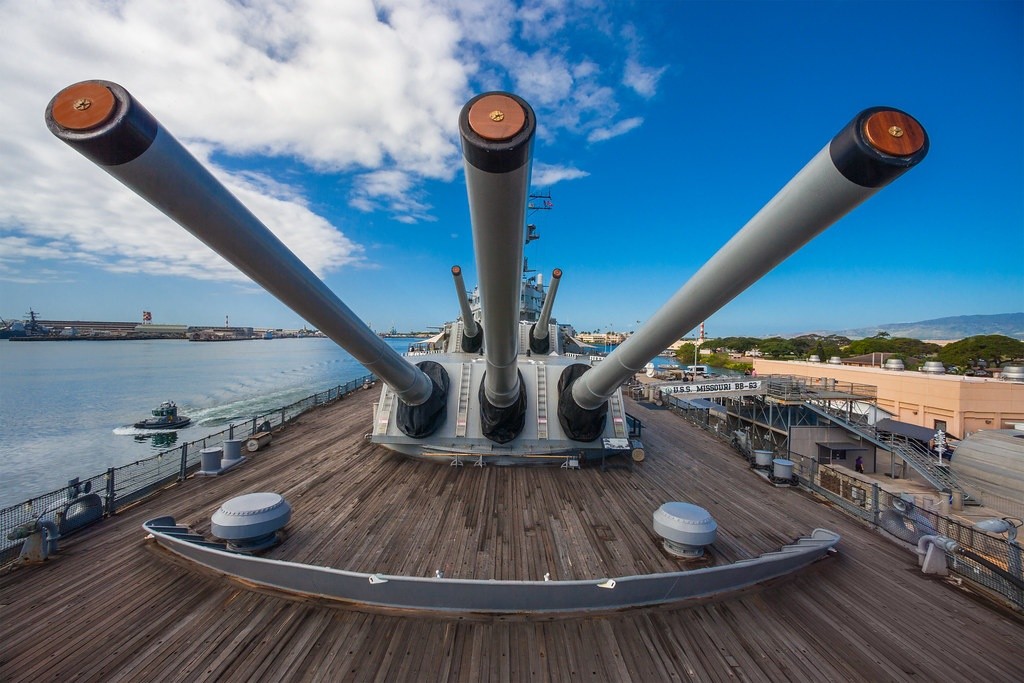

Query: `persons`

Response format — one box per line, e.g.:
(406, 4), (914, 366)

(854, 456), (864, 474)
(752, 368), (758, 378)
(682, 372), (694, 384)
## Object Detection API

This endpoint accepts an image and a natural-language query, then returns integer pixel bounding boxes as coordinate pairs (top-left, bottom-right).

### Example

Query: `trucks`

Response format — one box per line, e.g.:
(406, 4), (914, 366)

(684, 366), (707, 375)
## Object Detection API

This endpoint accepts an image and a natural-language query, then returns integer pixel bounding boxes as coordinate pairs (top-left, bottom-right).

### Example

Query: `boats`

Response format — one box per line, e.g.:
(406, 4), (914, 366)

(132, 400), (191, 430)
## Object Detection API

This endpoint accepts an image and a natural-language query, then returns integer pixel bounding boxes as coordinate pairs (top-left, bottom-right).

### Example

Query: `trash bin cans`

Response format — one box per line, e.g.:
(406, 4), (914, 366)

(895, 462), (903, 477)
